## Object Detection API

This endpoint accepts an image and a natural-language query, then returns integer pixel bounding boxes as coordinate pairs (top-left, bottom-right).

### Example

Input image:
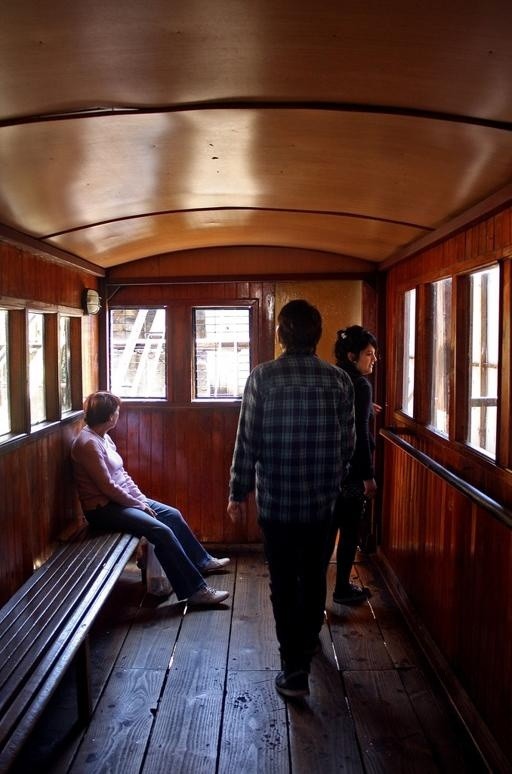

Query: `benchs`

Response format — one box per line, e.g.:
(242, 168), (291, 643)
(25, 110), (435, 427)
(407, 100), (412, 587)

(0, 529), (139, 769)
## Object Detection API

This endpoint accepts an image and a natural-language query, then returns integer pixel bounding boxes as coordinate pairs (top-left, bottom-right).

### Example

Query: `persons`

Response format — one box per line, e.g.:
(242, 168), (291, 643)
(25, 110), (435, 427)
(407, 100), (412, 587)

(226, 300), (358, 697)
(332, 325), (382, 606)
(68, 392), (230, 606)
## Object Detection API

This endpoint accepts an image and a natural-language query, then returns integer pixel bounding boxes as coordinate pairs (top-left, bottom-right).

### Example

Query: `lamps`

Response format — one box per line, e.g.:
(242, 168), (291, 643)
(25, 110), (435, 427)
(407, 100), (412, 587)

(82, 287), (102, 315)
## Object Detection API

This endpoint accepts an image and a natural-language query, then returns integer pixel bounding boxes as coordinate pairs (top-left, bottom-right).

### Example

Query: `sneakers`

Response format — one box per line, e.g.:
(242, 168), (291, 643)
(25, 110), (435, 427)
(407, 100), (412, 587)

(204, 557), (231, 570)
(333, 588), (368, 606)
(274, 671), (309, 697)
(189, 589), (230, 605)
